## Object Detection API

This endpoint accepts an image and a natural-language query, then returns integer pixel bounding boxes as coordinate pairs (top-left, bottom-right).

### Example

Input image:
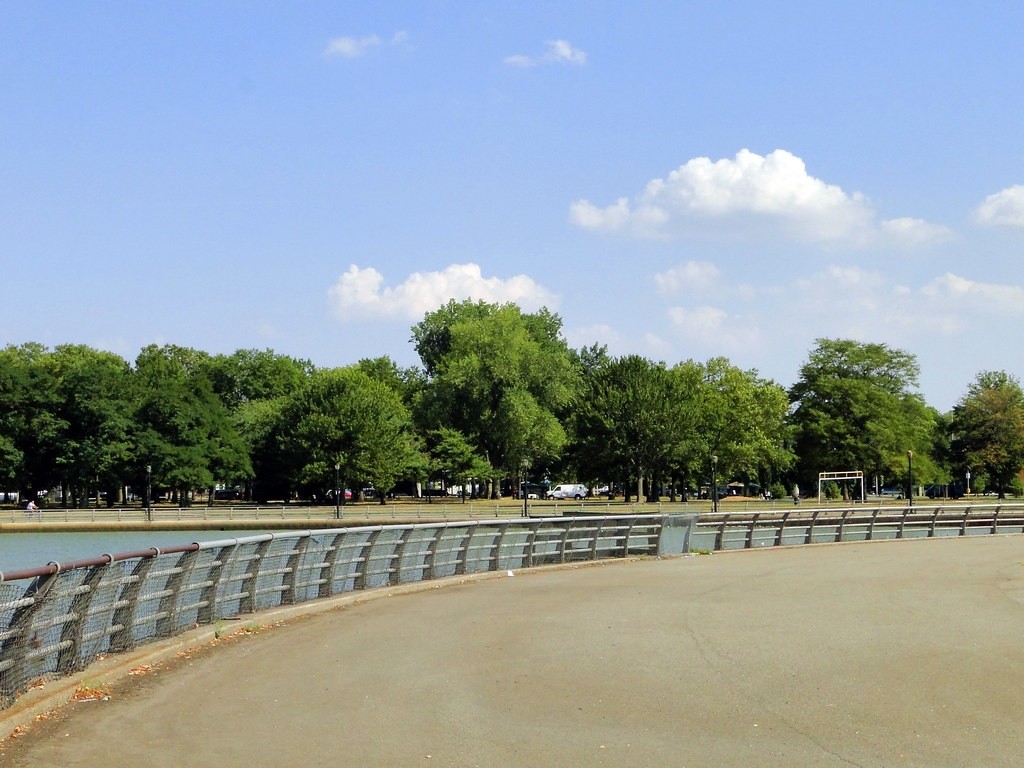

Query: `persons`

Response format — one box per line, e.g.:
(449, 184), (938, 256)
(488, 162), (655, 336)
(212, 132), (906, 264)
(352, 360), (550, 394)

(27, 500), (38, 510)
(792, 483), (800, 505)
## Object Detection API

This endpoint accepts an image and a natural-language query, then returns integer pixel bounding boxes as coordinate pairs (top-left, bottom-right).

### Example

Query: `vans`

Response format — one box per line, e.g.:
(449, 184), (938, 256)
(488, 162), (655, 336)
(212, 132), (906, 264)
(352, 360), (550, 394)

(546, 484), (589, 501)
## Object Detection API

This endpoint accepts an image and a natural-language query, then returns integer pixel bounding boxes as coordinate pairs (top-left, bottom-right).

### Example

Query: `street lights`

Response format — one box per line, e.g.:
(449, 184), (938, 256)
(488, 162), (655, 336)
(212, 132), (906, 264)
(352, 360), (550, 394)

(334, 464), (340, 518)
(523, 459), (529, 517)
(146, 465), (152, 522)
(712, 455), (719, 513)
(906, 450), (914, 515)
